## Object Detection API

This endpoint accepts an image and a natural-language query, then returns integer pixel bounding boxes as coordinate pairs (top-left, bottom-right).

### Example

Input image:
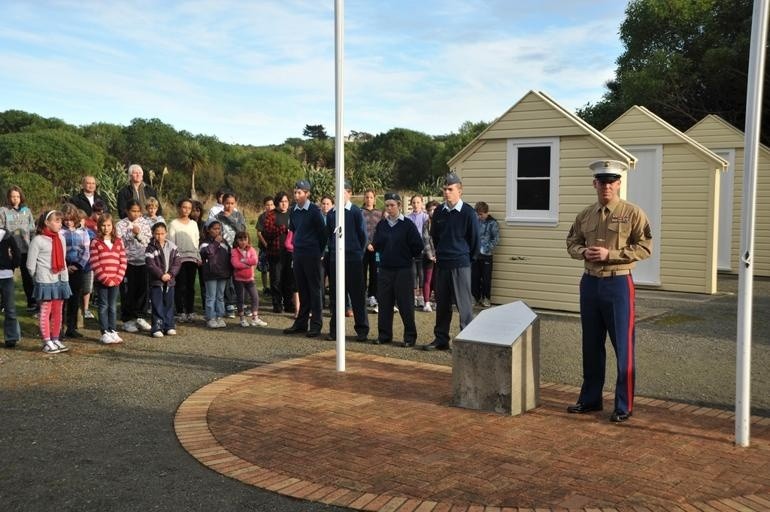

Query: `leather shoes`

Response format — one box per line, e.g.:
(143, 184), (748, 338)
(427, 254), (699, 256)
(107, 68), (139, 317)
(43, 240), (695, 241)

(566, 403), (604, 413)
(283, 327), (307, 334)
(307, 328), (322, 337)
(610, 411), (630, 421)
(423, 341), (450, 351)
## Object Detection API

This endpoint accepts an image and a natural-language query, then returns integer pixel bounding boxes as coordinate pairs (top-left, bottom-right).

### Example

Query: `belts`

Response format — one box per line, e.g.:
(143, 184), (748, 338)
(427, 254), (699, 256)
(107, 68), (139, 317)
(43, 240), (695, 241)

(585, 269), (631, 277)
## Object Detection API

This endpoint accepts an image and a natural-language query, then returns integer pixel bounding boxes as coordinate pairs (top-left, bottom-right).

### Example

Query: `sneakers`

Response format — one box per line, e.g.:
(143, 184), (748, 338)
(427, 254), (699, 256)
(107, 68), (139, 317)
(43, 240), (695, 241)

(5, 302), (268, 350)
(373, 339), (386, 345)
(357, 335), (368, 342)
(262, 286), (332, 319)
(347, 296), (492, 318)
(325, 333), (336, 340)
(402, 342), (416, 346)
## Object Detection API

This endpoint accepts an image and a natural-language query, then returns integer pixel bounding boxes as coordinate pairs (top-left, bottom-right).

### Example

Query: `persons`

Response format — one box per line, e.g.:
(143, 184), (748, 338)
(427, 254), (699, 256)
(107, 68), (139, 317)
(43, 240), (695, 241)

(471, 200), (501, 307)
(565, 159), (653, 422)
(256, 180), (441, 348)
(420, 173), (481, 350)
(1, 163), (267, 349)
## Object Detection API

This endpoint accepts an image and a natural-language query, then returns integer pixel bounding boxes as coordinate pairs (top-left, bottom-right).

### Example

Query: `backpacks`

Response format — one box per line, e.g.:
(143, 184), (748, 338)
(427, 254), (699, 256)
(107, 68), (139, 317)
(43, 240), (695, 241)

(0, 226), (22, 271)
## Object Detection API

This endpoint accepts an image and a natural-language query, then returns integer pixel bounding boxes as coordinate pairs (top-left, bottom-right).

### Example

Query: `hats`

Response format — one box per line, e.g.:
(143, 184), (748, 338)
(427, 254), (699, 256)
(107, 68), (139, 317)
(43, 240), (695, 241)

(384, 193), (401, 201)
(588, 160), (628, 183)
(295, 180), (310, 190)
(445, 172), (462, 185)
(344, 182), (352, 189)
